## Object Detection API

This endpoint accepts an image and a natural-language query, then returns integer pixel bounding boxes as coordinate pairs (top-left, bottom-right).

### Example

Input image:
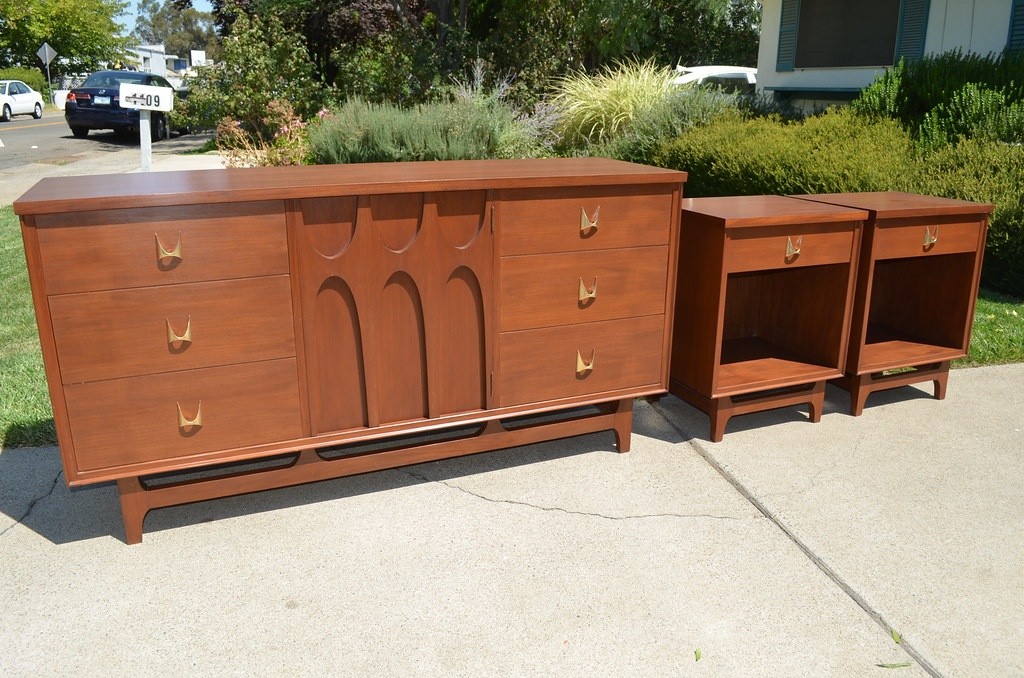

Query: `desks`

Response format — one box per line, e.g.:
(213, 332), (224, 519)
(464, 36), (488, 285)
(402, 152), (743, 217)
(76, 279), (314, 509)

(785, 191), (996, 412)
(643, 195), (870, 443)
(10, 155), (688, 545)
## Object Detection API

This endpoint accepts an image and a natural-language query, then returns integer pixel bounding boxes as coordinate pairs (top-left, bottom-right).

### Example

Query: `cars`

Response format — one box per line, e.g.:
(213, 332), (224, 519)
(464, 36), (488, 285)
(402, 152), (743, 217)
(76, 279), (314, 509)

(65, 70), (179, 141)
(0, 80), (46, 122)
(669, 64), (757, 100)
